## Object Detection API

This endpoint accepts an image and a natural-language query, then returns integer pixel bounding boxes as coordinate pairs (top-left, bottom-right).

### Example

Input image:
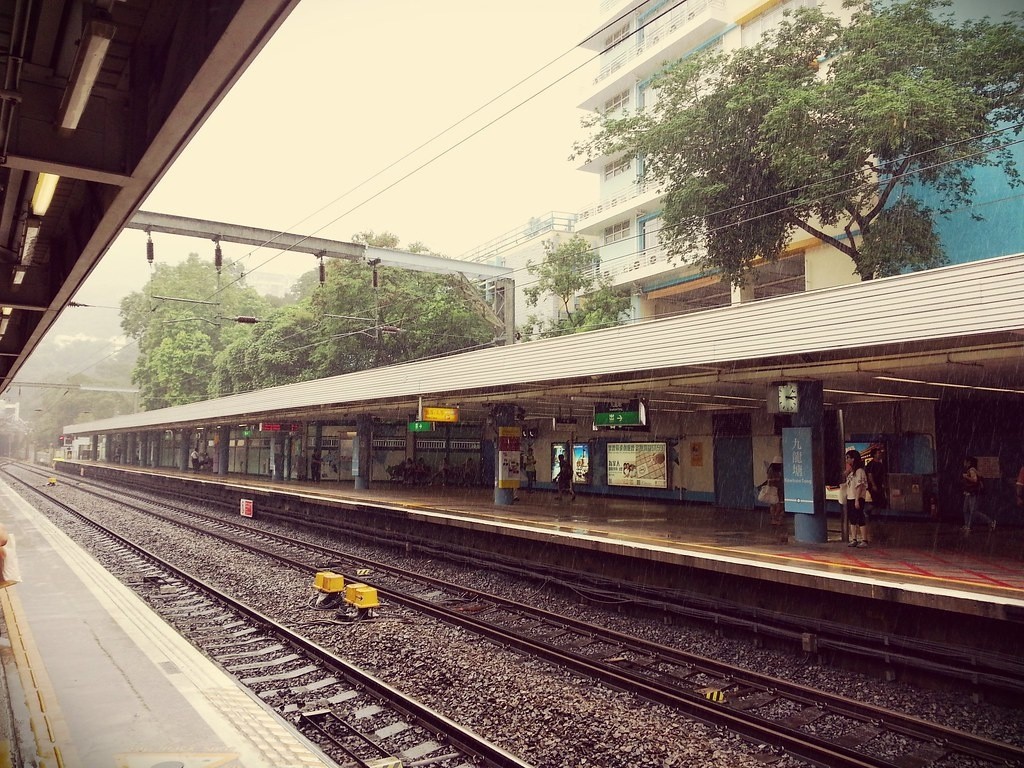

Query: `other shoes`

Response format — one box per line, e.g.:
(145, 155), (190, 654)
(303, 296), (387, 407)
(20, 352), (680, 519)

(554, 496), (563, 500)
(847, 541), (858, 547)
(856, 541), (868, 548)
(961, 526), (971, 530)
(570, 492), (576, 500)
(991, 519), (996, 531)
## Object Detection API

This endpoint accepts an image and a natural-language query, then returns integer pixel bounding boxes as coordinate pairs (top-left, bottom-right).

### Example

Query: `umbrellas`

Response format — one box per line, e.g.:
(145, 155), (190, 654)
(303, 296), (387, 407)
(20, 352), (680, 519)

(938, 463), (970, 501)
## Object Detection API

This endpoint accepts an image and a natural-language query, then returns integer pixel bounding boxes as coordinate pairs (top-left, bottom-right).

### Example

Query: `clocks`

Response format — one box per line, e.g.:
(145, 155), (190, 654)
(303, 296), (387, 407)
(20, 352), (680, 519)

(779, 382), (800, 413)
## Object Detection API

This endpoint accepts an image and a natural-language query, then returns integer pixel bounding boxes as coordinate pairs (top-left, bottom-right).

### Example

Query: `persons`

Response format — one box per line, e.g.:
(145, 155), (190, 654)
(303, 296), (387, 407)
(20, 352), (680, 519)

(866, 448), (886, 522)
(554, 455), (575, 501)
(523, 448), (536, 493)
(960, 458), (996, 531)
(310, 447), (321, 484)
(192, 448), (213, 474)
(766, 456), (784, 525)
(845, 450), (869, 547)
(402, 455), (474, 489)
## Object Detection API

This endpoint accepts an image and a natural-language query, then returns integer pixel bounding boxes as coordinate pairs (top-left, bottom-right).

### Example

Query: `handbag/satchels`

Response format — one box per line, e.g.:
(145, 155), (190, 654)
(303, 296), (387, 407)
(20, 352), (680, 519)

(838, 472), (851, 505)
(962, 468), (987, 496)
(758, 479), (780, 505)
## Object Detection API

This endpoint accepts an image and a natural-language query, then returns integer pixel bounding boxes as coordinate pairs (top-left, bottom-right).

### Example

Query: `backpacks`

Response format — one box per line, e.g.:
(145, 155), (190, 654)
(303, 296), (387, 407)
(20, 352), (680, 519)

(561, 463), (573, 480)
